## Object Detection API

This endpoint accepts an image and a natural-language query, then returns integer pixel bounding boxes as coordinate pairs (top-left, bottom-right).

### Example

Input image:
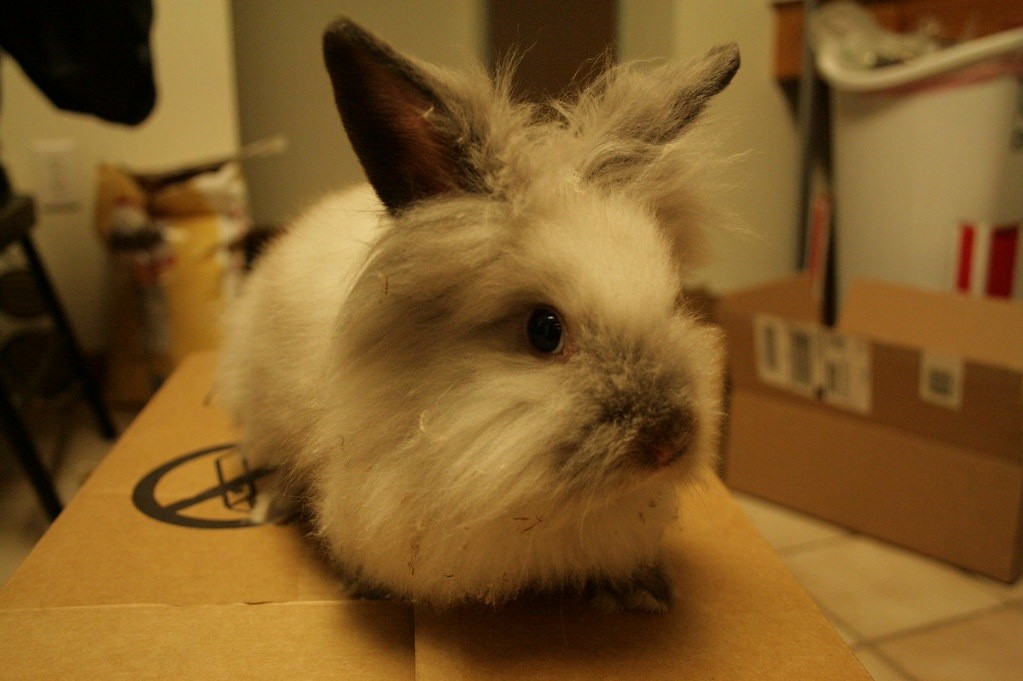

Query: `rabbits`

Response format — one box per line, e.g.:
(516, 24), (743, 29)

(217, 24), (752, 610)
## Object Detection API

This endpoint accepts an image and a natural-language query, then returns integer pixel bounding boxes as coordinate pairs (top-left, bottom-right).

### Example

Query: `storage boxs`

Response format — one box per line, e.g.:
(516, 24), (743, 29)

(0, 350), (882, 681)
(688, 275), (1023, 585)
